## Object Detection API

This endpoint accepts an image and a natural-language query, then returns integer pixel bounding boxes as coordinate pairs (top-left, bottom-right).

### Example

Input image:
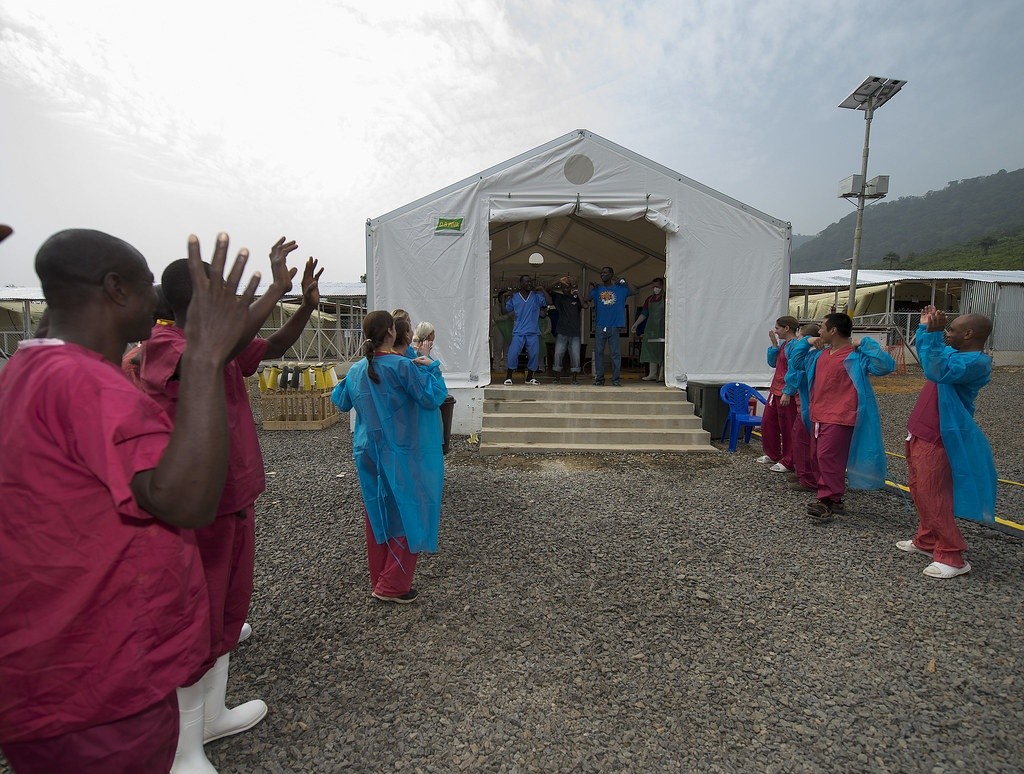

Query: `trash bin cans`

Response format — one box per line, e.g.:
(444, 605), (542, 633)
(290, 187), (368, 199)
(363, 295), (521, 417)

(440, 395), (456, 455)
(687, 380), (744, 440)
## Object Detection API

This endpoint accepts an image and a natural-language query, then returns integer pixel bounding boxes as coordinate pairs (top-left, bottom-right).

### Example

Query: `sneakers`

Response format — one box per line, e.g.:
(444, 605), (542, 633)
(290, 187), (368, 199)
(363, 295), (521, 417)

(371, 588), (419, 604)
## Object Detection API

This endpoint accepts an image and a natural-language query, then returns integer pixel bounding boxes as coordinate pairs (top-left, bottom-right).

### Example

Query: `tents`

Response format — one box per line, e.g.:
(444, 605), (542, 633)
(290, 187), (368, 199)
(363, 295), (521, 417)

(365, 128), (793, 387)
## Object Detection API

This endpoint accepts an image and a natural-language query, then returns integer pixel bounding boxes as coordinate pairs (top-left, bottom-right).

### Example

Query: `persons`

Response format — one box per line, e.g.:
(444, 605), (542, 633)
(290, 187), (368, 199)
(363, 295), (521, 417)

(140, 237), (325, 774)
(501, 275), (549, 386)
(0, 230), (262, 774)
(894, 305), (993, 579)
(392, 309), (435, 361)
(792, 313), (896, 518)
(755, 315), (801, 472)
(631, 278), (665, 382)
(332, 311), (447, 602)
(125, 284), (254, 644)
(787, 324), (821, 491)
(546, 277), (587, 386)
(584, 266), (630, 386)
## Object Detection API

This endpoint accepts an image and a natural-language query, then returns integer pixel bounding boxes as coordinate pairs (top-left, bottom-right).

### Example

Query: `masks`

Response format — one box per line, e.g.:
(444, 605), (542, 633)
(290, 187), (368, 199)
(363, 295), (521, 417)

(654, 287), (661, 294)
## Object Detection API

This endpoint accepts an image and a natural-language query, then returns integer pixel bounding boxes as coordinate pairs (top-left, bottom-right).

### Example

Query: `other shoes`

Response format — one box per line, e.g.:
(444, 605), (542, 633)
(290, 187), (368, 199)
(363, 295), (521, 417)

(790, 482), (812, 491)
(593, 382), (604, 386)
(805, 498), (834, 521)
(787, 473), (799, 482)
(552, 380), (561, 384)
(571, 381), (580, 386)
(238, 623), (251, 643)
(806, 499), (844, 514)
(614, 381), (621, 386)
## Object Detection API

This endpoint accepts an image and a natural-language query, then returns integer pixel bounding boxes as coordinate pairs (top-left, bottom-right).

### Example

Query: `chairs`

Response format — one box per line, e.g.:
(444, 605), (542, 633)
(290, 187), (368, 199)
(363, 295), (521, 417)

(720, 383), (767, 452)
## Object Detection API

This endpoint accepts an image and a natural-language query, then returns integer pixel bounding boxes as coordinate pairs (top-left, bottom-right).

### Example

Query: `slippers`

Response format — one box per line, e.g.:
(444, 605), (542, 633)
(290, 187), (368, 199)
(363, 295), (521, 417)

(769, 463), (791, 472)
(525, 379), (540, 385)
(503, 379), (513, 386)
(757, 455), (777, 463)
(922, 560), (971, 578)
(896, 540), (933, 558)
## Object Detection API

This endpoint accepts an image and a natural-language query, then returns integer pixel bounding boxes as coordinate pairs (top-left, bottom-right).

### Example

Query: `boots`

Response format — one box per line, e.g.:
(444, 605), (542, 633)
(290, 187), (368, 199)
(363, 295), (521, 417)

(658, 362), (664, 381)
(642, 363), (658, 380)
(170, 677), (219, 774)
(203, 653), (268, 745)
